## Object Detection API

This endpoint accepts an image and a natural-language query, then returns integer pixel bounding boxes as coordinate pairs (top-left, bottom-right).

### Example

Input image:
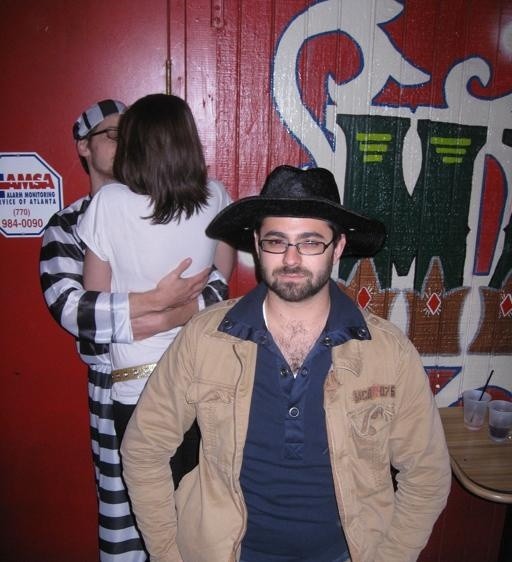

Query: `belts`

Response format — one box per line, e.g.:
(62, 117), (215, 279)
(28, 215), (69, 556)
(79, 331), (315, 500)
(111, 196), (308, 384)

(110, 362), (158, 383)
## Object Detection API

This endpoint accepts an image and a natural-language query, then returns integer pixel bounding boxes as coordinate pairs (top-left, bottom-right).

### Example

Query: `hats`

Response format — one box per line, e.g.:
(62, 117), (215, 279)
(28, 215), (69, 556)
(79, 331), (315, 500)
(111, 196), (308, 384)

(73, 99), (129, 140)
(205, 165), (385, 258)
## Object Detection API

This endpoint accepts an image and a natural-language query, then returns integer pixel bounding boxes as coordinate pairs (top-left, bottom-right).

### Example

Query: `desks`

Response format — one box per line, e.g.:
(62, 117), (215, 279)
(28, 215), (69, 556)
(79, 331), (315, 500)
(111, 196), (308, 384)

(439, 407), (511, 562)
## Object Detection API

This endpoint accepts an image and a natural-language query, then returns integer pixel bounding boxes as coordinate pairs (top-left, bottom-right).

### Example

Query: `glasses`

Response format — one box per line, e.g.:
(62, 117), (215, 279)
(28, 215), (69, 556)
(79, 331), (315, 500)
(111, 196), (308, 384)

(80, 129), (119, 141)
(257, 239), (333, 255)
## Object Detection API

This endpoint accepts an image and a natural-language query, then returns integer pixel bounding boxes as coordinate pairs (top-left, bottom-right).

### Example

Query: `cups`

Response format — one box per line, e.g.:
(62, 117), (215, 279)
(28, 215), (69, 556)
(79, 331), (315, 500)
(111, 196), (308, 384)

(462, 390), (492, 432)
(486, 400), (512, 442)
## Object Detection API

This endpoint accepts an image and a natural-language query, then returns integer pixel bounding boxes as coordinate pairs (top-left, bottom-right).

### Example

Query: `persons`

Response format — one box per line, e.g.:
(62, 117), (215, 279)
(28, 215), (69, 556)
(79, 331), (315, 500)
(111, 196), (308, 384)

(71, 91), (239, 560)
(38, 96), (237, 561)
(114, 161), (457, 561)
